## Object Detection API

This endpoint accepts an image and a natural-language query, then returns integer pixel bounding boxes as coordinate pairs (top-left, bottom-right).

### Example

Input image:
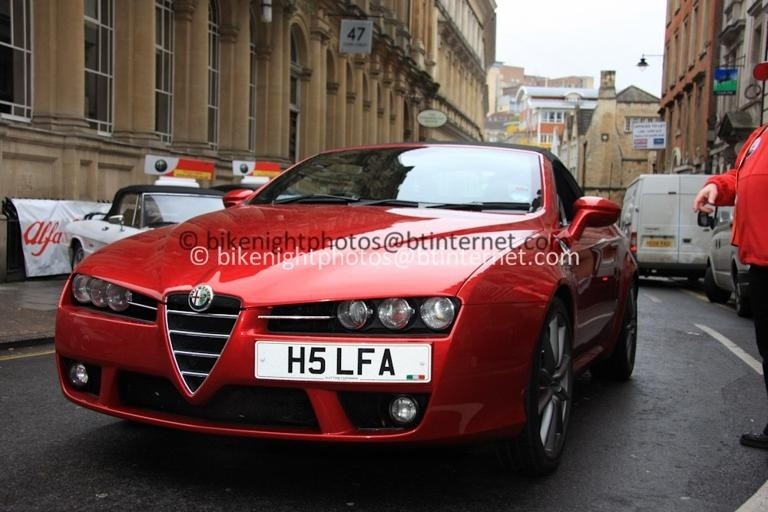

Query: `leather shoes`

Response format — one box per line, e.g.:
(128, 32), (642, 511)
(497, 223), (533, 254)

(740, 431), (768, 449)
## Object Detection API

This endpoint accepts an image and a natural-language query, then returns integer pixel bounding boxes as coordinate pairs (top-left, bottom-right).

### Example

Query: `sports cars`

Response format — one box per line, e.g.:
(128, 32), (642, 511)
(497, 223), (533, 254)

(46, 138), (646, 480)
(60, 181), (229, 274)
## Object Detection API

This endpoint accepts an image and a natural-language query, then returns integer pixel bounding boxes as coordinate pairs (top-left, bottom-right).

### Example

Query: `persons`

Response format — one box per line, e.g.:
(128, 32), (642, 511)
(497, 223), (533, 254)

(693, 125), (764, 450)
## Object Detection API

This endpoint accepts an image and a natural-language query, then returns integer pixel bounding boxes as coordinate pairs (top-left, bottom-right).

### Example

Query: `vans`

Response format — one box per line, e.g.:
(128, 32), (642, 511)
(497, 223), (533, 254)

(696, 196), (750, 317)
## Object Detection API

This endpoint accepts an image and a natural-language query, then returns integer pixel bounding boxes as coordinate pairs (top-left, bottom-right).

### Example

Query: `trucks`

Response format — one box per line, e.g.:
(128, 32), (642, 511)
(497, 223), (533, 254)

(613, 169), (722, 286)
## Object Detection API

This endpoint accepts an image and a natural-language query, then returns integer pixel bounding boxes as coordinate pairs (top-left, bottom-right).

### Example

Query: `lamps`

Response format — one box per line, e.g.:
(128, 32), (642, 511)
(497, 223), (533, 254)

(637, 52), (664, 66)
